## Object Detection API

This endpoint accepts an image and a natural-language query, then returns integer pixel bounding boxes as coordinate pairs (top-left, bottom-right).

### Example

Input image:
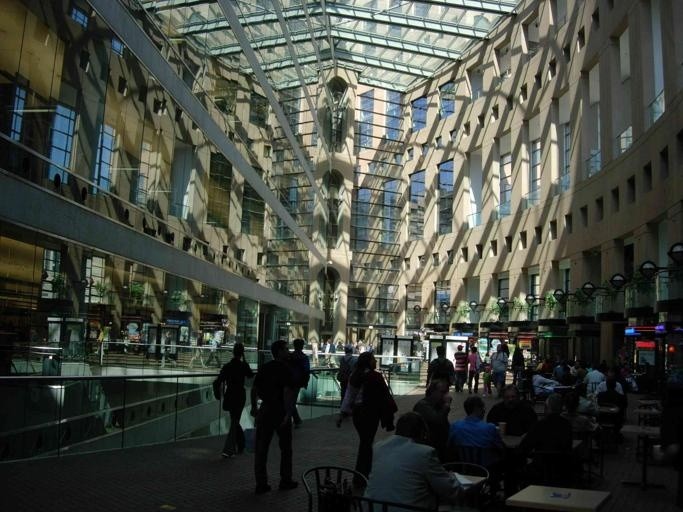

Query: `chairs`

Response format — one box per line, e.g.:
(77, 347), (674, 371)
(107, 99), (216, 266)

(520, 378), (626, 440)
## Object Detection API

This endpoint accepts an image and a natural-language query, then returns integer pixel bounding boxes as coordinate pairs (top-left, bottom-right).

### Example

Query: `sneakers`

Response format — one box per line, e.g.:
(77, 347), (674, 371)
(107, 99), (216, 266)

(221, 447), (237, 458)
(255, 485), (270, 495)
(279, 479), (298, 491)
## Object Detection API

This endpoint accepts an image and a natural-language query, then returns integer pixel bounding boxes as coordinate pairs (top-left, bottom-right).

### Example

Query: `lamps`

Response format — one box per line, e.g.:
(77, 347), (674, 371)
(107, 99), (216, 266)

(414, 242), (683, 314)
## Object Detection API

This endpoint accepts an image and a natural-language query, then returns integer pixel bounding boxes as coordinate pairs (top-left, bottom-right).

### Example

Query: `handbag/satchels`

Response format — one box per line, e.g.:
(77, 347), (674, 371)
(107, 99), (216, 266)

(337, 356), (352, 383)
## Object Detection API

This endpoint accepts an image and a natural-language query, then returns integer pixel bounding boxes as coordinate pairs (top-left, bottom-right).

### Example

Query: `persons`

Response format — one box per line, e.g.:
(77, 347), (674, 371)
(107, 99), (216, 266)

(189, 333), (204, 367)
(213, 343), (254, 458)
(205, 335), (221, 368)
(289, 339), (311, 425)
(320, 339), (375, 368)
(336, 352), (398, 504)
(160, 333), (177, 368)
(339, 345), (355, 405)
(250, 340), (298, 495)
(414, 345), (672, 491)
(94, 328), (105, 355)
(360, 412), (465, 512)
